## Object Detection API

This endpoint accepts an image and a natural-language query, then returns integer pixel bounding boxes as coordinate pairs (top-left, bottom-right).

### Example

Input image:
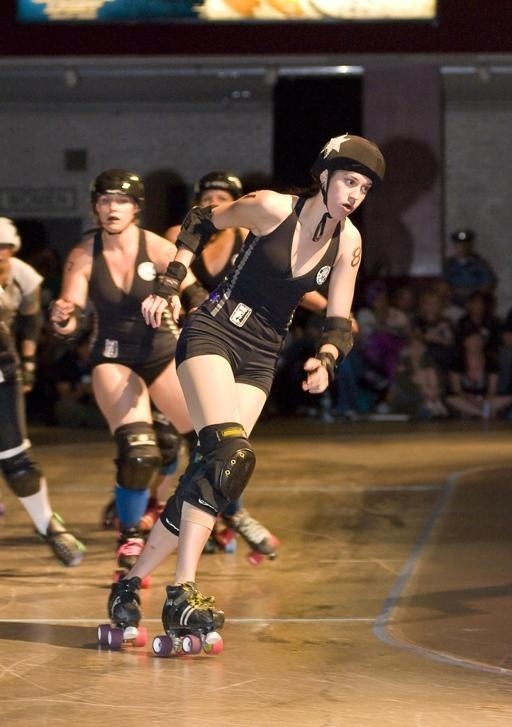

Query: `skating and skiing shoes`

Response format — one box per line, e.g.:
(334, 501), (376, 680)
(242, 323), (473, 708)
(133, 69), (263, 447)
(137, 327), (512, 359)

(101, 498), (280, 571)
(36, 515), (87, 567)
(96, 580), (226, 657)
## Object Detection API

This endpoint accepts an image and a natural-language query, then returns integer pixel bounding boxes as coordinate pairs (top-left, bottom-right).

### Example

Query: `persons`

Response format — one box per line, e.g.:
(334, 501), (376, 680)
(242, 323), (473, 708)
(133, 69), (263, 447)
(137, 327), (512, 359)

(50, 167), (281, 587)
(98, 134), (385, 660)
(105, 172), (361, 553)
(0, 214), (44, 512)
(1, 314), (87, 565)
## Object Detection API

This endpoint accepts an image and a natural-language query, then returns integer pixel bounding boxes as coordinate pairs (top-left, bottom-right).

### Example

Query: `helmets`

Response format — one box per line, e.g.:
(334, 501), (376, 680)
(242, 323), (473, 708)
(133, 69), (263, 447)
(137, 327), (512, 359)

(192, 169), (242, 202)
(90, 168), (145, 211)
(316, 134), (386, 185)
(0, 217), (22, 254)
